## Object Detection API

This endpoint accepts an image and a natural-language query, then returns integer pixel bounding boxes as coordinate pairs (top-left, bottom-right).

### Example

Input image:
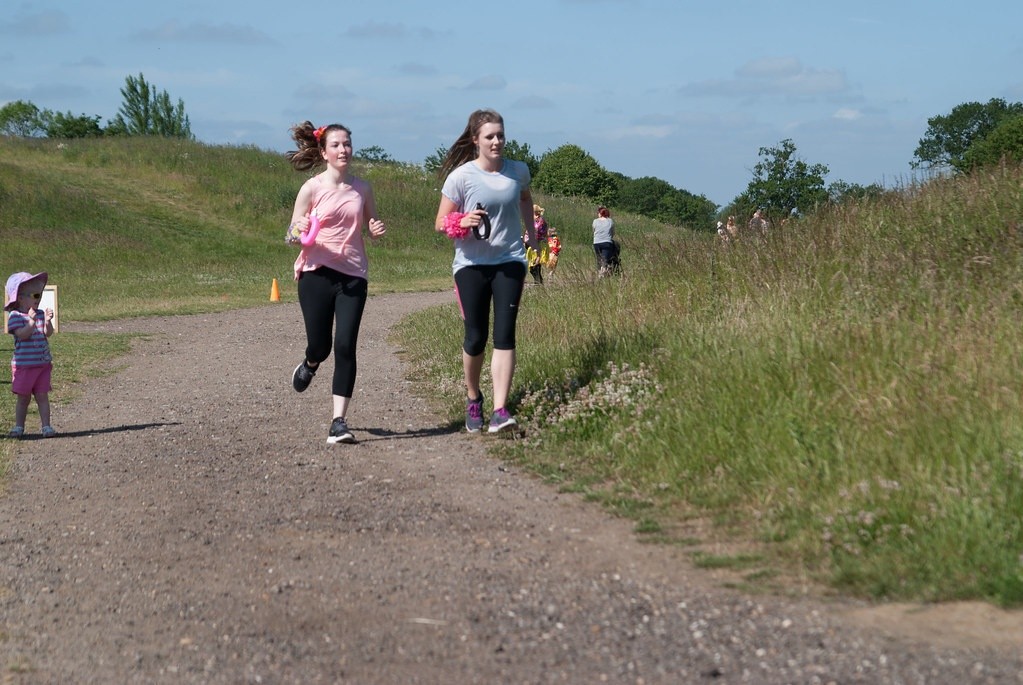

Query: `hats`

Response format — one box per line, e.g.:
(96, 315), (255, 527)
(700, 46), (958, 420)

(4, 271), (48, 311)
(717, 221), (723, 228)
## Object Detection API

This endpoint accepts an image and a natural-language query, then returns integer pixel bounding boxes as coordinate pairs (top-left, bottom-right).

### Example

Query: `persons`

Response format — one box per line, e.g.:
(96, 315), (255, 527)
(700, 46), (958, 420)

(717, 207), (805, 243)
(435, 109), (540, 434)
(284, 121), (387, 443)
(592, 207), (615, 280)
(3, 271), (55, 437)
(524, 204), (561, 286)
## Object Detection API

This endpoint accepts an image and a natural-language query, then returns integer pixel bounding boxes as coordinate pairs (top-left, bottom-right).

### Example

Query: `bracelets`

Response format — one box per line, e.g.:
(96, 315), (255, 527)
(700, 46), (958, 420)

(285, 221), (302, 245)
(443, 212), (471, 240)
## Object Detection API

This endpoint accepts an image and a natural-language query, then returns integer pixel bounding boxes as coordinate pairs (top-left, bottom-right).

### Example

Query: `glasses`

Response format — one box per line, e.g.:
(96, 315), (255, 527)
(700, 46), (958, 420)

(19, 292), (40, 299)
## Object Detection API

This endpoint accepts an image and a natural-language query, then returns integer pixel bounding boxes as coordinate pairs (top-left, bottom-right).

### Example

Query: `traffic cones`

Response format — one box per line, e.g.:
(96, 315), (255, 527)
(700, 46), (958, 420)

(269, 275), (282, 303)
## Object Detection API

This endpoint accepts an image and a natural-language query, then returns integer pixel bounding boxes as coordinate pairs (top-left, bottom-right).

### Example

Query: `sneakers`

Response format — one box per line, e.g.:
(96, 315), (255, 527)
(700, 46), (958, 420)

(465, 389), (484, 433)
(326, 417), (355, 443)
(488, 407), (518, 433)
(292, 356), (319, 393)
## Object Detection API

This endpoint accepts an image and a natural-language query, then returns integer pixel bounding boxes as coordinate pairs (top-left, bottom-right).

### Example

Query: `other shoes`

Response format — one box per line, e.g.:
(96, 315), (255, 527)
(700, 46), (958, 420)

(41, 426), (55, 437)
(10, 426), (24, 438)
